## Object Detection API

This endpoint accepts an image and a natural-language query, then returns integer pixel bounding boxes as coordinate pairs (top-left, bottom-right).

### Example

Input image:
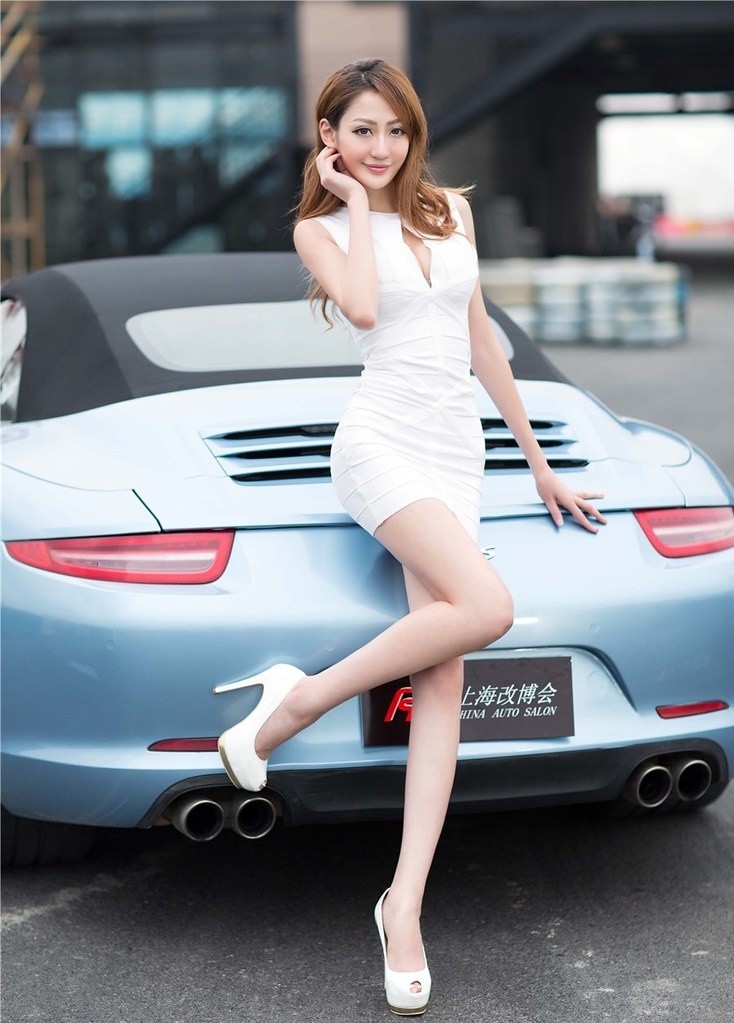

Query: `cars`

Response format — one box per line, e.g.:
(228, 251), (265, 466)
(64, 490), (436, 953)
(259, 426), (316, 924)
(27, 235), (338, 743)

(0, 249), (734, 870)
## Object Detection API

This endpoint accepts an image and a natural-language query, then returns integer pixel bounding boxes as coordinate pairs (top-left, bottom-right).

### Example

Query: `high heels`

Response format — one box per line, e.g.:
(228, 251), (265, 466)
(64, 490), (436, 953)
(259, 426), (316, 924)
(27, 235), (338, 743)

(214, 661), (306, 793)
(373, 887), (431, 1015)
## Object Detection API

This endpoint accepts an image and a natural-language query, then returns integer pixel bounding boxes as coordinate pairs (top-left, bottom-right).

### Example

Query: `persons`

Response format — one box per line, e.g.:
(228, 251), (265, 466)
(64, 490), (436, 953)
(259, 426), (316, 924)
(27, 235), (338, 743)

(212, 58), (607, 1017)
